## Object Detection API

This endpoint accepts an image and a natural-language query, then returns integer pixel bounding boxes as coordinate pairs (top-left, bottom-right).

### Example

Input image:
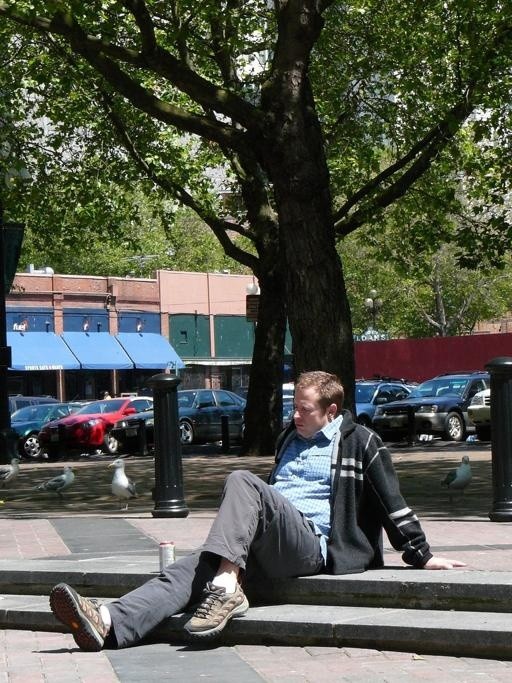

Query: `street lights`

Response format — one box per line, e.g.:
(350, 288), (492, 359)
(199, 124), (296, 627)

(243, 276), (258, 340)
(362, 289), (383, 341)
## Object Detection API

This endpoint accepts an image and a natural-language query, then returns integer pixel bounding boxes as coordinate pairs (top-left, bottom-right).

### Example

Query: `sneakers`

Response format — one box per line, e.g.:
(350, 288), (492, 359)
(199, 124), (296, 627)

(48, 582), (111, 652)
(183, 580), (251, 639)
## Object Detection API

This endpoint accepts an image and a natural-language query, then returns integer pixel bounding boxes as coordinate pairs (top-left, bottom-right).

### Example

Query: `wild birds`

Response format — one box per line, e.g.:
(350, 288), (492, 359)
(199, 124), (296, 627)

(107, 458), (140, 511)
(0, 458), (24, 489)
(35, 464), (81, 500)
(441, 455), (473, 497)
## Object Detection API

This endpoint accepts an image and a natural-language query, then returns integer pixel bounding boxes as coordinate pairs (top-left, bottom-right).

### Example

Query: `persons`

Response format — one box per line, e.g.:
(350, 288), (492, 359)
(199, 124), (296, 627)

(104, 391), (111, 400)
(49, 371), (467, 652)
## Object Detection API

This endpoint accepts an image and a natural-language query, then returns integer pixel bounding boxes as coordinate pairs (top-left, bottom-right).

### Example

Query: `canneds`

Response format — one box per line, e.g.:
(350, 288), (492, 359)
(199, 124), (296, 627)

(159, 541), (175, 572)
